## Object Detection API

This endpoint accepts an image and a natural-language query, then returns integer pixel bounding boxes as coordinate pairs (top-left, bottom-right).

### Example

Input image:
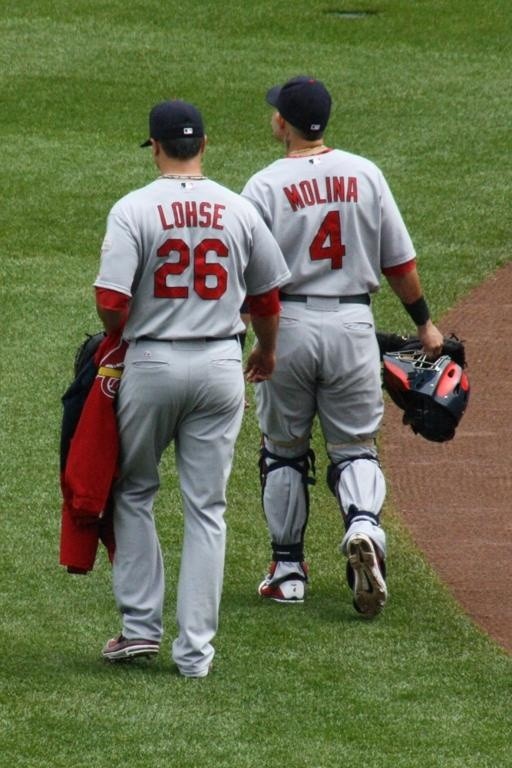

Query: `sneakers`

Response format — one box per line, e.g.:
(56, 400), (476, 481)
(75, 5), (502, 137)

(257, 576), (307, 605)
(345, 533), (388, 619)
(100, 635), (161, 663)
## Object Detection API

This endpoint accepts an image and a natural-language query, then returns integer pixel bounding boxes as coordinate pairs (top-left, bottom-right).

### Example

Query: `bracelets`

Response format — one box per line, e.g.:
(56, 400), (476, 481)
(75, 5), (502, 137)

(239, 333), (246, 351)
(401, 296), (431, 325)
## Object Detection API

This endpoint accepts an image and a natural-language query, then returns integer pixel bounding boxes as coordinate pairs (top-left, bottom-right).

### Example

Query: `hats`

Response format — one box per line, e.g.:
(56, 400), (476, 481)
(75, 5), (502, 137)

(265, 73), (333, 134)
(138, 100), (206, 150)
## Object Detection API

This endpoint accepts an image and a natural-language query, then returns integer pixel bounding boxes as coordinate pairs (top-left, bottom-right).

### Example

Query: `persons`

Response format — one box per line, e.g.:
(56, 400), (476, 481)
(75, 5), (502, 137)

(239, 78), (444, 618)
(94, 100), (292, 677)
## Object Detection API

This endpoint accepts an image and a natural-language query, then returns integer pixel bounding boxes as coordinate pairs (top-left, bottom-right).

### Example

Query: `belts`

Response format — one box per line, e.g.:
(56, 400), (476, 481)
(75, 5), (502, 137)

(137, 334), (240, 343)
(276, 289), (372, 306)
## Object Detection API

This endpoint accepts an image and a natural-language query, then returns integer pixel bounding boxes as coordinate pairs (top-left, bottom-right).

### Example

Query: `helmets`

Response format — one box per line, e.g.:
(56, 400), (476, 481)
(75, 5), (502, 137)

(379, 351), (471, 428)
(72, 330), (109, 376)
